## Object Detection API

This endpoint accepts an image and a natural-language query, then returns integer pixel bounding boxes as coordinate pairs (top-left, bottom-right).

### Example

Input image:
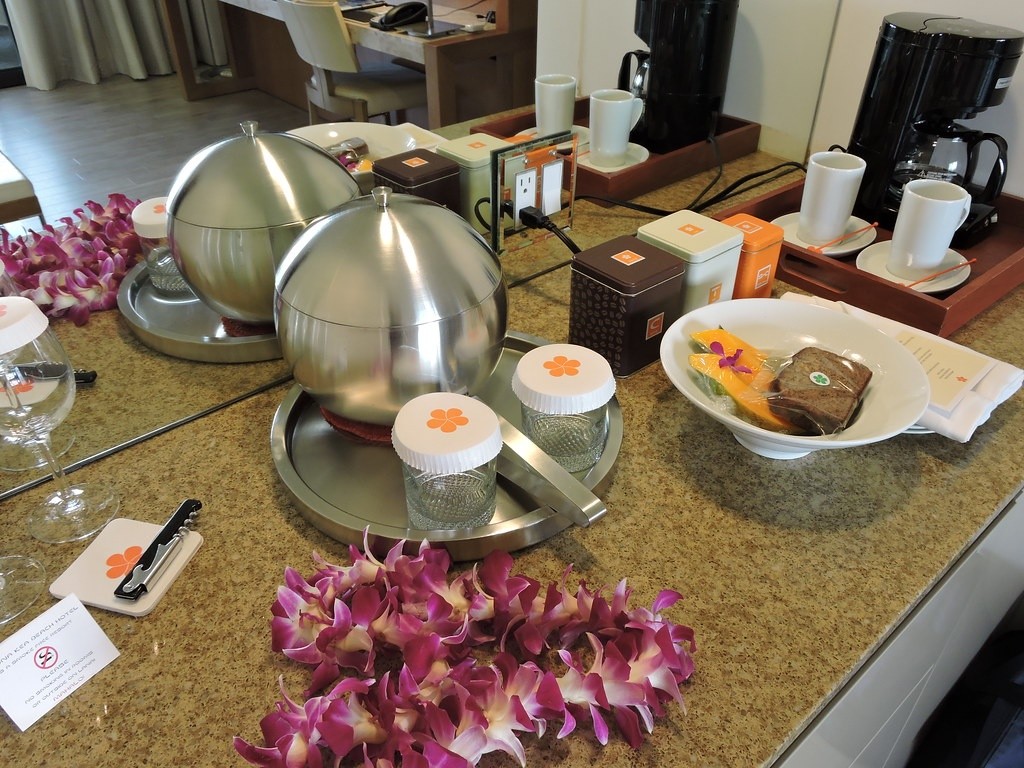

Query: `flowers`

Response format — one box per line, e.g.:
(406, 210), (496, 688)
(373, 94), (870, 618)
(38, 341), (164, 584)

(222, 521), (699, 768)
(0, 192), (161, 328)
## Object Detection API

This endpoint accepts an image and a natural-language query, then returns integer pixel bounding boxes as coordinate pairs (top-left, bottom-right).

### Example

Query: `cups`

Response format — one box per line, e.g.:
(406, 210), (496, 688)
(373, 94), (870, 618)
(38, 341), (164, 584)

(131, 196), (191, 296)
(797, 151), (866, 247)
(886, 179), (971, 281)
(464, 24), (484, 31)
(391, 392), (502, 530)
(535, 74), (576, 136)
(512, 344), (616, 473)
(590, 90), (643, 165)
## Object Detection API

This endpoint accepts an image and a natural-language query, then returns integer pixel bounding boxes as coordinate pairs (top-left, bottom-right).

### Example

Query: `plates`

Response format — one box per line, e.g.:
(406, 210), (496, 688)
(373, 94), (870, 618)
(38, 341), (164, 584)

(856, 240), (971, 292)
(460, 28), (475, 32)
(514, 125), (589, 150)
(903, 424), (934, 435)
(770, 212), (876, 254)
(571, 142), (650, 173)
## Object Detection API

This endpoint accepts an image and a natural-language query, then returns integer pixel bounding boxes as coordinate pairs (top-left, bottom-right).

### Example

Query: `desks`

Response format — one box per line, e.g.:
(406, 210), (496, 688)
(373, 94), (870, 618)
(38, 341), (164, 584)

(158, 0), (537, 128)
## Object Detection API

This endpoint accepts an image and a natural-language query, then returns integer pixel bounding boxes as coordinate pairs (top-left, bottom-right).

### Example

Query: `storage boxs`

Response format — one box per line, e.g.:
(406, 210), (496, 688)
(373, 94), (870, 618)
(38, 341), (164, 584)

(467, 93), (762, 211)
(637, 207), (745, 314)
(435, 131), (514, 237)
(710, 175), (1024, 340)
(372, 148), (462, 217)
(720, 212), (785, 301)
(566, 234), (685, 380)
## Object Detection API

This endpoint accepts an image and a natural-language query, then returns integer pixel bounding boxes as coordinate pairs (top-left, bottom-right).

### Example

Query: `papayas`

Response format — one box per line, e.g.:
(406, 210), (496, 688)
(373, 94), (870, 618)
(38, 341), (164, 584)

(688, 327), (809, 436)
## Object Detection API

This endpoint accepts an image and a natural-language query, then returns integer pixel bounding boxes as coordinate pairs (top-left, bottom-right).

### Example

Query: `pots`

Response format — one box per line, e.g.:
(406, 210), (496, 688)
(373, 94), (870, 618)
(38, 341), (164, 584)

(274, 186), (508, 426)
(169, 121), (365, 322)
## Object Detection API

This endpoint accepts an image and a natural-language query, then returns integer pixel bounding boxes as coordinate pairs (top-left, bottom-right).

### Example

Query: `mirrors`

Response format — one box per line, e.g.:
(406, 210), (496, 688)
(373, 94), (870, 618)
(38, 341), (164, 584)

(0, 0), (844, 506)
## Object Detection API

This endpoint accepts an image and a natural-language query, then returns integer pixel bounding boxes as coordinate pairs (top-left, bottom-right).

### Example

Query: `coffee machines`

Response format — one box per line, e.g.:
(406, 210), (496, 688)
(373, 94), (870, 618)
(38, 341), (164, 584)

(618, 0), (739, 156)
(846, 12), (1024, 248)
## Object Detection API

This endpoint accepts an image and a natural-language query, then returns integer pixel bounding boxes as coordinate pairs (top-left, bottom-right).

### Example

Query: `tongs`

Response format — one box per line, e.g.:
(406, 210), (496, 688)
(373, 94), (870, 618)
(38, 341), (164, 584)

(468, 394), (606, 526)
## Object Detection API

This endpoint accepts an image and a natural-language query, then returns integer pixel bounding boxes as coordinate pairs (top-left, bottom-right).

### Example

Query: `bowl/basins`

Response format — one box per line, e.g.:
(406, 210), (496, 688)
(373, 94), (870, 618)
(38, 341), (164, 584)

(286, 122), (448, 197)
(660, 298), (930, 460)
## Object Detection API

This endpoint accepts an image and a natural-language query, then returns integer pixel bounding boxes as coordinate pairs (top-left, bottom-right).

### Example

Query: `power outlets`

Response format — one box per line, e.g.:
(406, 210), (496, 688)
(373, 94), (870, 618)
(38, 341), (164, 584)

(493, 135), (579, 253)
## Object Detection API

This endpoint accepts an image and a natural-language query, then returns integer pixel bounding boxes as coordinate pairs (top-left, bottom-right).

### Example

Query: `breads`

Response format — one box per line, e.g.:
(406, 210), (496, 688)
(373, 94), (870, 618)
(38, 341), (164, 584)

(765, 347), (872, 435)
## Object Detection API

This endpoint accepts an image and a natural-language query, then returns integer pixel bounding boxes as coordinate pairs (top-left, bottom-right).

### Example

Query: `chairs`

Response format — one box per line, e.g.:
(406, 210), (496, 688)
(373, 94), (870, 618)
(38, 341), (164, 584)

(275, 0), (427, 127)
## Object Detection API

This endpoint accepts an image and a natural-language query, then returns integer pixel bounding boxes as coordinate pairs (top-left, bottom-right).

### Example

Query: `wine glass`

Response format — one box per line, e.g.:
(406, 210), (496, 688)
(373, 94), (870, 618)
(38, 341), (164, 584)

(0, 554), (46, 625)
(0, 348), (75, 471)
(0, 326), (121, 544)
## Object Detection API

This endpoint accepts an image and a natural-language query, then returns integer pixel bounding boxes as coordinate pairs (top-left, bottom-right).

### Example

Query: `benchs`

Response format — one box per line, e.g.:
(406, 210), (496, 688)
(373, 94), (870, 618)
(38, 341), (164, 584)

(0, 150), (49, 232)
